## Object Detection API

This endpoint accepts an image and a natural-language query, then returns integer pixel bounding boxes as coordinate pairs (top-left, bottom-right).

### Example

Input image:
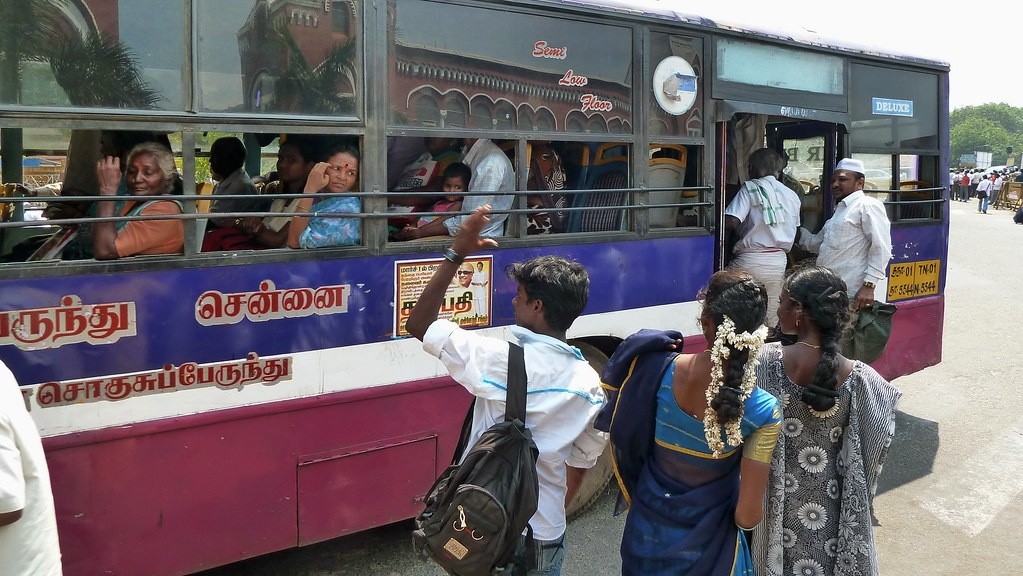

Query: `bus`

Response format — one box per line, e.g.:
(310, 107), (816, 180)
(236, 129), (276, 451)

(0, 0), (951, 576)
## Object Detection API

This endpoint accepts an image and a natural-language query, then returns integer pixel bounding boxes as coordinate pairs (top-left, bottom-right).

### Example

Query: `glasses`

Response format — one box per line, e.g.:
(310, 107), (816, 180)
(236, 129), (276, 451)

(458, 271), (474, 275)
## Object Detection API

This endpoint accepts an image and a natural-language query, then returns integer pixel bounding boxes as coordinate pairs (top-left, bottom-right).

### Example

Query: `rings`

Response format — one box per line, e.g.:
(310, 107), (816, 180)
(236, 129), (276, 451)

(865, 304), (870, 307)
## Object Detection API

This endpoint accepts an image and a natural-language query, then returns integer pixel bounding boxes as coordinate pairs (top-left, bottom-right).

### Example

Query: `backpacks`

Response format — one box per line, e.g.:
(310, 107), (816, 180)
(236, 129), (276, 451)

(411, 342), (539, 576)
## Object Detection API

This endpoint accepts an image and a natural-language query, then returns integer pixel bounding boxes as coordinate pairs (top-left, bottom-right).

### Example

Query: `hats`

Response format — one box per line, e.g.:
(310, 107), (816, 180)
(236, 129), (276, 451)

(835, 157), (865, 175)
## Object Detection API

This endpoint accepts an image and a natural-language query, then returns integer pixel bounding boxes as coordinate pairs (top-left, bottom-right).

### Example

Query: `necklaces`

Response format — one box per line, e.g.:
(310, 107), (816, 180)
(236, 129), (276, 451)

(795, 342), (820, 349)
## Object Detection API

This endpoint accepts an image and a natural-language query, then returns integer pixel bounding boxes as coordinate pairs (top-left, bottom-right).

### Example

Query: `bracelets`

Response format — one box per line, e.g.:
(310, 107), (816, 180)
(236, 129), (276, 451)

(864, 282), (875, 288)
(443, 248), (463, 264)
(296, 206), (311, 212)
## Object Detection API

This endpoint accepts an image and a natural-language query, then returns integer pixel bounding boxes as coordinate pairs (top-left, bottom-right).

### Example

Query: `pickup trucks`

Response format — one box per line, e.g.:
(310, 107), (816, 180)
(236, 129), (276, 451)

(798, 168), (823, 185)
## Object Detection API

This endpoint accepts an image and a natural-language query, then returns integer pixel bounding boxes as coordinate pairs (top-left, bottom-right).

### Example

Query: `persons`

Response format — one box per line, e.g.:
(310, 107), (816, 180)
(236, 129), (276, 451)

(0, 360), (63, 576)
(527, 140), (569, 234)
(471, 262), (488, 316)
(725, 148), (801, 328)
(405, 205), (610, 576)
(793, 158), (891, 310)
(726, 113), (768, 209)
(782, 150), (805, 196)
(949, 167), (1023, 205)
(976, 174), (992, 213)
(751, 266), (903, 576)
(387, 136), (515, 238)
(206, 134), (361, 249)
(620, 268), (783, 576)
(458, 262), (474, 287)
(17, 130), (184, 261)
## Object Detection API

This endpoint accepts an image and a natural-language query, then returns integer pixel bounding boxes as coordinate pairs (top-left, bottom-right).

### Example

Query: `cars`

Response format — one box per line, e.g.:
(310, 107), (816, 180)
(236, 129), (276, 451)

(864, 169), (892, 190)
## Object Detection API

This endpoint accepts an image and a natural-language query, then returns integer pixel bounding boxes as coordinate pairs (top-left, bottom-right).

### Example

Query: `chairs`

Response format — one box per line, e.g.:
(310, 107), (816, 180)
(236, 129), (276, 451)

(27, 142), (934, 264)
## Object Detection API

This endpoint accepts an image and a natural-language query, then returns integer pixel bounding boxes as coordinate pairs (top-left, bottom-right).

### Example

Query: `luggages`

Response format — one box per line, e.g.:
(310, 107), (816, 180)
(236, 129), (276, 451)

(1013, 208), (1023, 225)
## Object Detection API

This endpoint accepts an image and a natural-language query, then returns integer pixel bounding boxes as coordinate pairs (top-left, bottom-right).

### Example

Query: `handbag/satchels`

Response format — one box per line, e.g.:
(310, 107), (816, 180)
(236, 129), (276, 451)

(836, 300), (897, 364)
(24, 224), (80, 261)
(977, 191), (987, 198)
(388, 152), (440, 228)
